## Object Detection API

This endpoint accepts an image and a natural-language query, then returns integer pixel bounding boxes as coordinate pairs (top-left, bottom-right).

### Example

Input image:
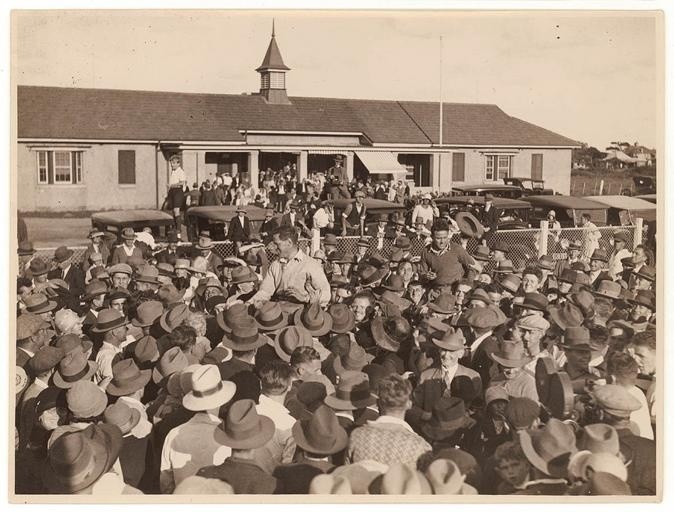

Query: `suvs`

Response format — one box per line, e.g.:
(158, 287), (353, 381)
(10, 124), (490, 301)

(85, 206), (179, 247)
(182, 204), (277, 241)
(436, 173), (656, 270)
(318, 195), (404, 236)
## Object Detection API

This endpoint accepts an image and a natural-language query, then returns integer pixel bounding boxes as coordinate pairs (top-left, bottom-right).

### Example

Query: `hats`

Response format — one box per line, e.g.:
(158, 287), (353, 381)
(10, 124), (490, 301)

(354, 191), (365, 198)
(378, 214), (426, 225)
(484, 194), (493, 202)
(17, 227), (656, 495)
(264, 209), (273, 217)
(456, 212), (484, 236)
(334, 155), (343, 162)
(290, 203), (299, 209)
(236, 205), (247, 214)
(422, 193), (432, 200)
